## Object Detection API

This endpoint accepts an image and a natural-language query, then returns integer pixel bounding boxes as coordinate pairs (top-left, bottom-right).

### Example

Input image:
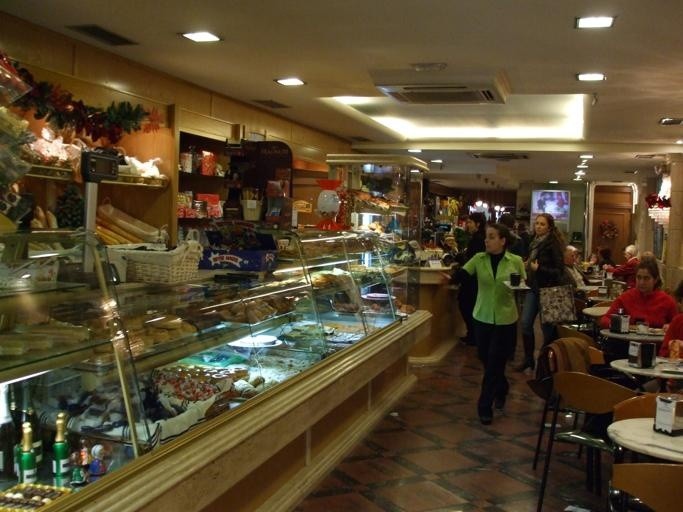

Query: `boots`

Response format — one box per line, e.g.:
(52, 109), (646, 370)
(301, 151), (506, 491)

(513, 333), (535, 371)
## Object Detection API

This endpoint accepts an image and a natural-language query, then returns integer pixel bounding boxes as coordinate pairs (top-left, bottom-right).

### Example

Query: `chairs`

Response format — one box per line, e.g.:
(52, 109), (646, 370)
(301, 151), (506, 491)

(538, 371), (645, 512)
(558, 324), (603, 349)
(609, 461), (683, 512)
(612, 393), (683, 421)
(533, 339), (606, 469)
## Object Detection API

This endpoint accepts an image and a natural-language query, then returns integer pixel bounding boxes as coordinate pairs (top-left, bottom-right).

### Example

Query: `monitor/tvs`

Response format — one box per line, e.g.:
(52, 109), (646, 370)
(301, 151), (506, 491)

(530, 189), (571, 224)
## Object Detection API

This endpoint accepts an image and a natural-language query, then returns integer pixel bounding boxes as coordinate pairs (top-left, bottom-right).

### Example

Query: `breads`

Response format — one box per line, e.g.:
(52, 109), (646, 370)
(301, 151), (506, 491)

(384, 264), (398, 273)
(93, 215), (145, 246)
(1, 263), (359, 366)
(95, 216), (144, 243)
(392, 299), (416, 313)
(384, 265), (399, 272)
(394, 300), (415, 313)
(96, 225), (133, 244)
(371, 303), (381, 311)
(371, 304), (380, 311)
(30, 206), (58, 229)
(45, 361), (265, 430)
(279, 237), (374, 259)
(95, 232), (120, 245)
(279, 237), (373, 259)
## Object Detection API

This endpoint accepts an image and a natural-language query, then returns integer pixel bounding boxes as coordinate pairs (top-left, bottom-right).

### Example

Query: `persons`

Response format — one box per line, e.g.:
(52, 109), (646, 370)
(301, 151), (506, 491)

(449, 223), (527, 425)
(449, 212), (529, 346)
(575, 250), (597, 270)
(599, 248), (614, 270)
(513, 214), (565, 373)
(563, 244), (589, 302)
(599, 258), (681, 365)
(634, 283), (682, 393)
(603, 245), (639, 289)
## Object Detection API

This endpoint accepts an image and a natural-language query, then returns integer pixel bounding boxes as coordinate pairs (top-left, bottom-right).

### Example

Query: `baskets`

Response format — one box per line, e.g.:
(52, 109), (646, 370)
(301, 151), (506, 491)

(118, 174), (168, 189)
(97, 196), (203, 284)
(327, 237), (373, 253)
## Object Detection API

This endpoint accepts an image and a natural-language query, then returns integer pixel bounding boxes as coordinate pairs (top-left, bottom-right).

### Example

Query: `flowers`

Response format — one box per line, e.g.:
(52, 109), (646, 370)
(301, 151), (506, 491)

(596, 219), (620, 241)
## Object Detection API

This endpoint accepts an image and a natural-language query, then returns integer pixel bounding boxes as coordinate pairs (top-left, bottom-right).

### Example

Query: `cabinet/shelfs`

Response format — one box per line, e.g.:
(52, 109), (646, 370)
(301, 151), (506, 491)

(1, 229), (432, 512)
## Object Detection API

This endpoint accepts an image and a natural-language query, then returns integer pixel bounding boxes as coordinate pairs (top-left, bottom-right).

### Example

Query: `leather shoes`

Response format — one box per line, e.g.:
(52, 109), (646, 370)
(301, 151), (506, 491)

(480, 413), (492, 425)
(494, 394), (506, 409)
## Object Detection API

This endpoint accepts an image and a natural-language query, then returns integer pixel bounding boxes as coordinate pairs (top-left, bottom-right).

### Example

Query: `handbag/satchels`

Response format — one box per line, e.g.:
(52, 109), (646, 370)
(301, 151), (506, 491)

(539, 284), (577, 325)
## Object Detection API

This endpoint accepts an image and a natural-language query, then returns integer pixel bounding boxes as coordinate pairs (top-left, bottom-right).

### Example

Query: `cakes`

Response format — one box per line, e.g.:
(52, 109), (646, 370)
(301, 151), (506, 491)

(30, 358), (269, 453)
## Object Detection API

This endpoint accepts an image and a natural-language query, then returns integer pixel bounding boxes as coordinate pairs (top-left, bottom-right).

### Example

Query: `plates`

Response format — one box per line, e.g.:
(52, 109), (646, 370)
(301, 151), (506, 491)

(658, 355), (682, 373)
(229, 334), (282, 349)
(629, 323), (667, 337)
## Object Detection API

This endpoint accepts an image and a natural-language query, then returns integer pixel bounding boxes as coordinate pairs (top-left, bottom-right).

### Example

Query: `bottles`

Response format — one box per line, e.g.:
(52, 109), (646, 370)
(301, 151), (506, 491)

(0, 381), (70, 480)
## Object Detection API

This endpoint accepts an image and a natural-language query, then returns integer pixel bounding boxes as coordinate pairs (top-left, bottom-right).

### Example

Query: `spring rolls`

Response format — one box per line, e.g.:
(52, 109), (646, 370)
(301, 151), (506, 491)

(45, 210), (58, 228)
(34, 206), (48, 228)
(32, 218), (44, 228)
